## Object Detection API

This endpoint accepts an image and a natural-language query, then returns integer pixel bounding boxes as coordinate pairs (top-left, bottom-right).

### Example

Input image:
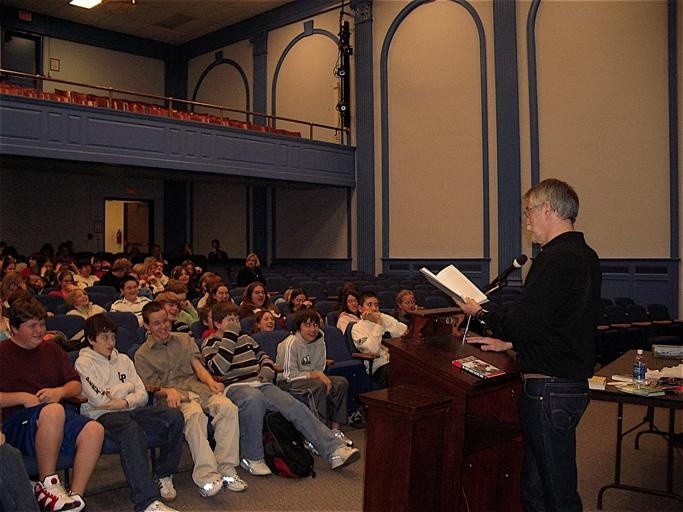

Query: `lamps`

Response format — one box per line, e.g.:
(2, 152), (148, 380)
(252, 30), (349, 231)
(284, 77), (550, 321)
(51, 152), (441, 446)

(337, 100), (347, 112)
(334, 64), (346, 78)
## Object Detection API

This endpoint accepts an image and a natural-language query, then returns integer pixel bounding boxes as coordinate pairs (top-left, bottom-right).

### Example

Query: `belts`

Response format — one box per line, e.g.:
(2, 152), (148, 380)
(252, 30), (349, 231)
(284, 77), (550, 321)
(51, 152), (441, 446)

(522, 371), (557, 380)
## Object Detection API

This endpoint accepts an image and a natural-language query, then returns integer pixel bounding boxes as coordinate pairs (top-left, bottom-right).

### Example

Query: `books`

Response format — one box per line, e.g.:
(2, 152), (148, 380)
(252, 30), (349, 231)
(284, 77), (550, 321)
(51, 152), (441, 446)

(618, 383), (666, 397)
(452, 357), (506, 380)
(419, 263), (490, 305)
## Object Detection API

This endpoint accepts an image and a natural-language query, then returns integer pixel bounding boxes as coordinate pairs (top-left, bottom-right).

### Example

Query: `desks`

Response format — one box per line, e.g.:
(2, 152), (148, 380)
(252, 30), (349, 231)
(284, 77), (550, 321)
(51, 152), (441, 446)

(587, 348), (683, 512)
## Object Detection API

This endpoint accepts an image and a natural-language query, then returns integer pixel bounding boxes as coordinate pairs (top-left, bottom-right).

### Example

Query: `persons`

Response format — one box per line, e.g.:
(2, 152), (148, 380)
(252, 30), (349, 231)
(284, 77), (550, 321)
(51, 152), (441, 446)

(451, 178), (602, 511)
(0, 239), (416, 512)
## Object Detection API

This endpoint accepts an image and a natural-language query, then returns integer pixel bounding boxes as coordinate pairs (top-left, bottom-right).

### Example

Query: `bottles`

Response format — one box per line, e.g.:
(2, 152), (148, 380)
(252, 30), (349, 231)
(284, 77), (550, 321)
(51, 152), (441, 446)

(633, 350), (646, 390)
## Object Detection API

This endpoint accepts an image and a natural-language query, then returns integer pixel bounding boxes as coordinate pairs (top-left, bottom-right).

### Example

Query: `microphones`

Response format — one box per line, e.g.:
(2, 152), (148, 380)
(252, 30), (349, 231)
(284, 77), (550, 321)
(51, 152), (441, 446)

(489, 254), (528, 288)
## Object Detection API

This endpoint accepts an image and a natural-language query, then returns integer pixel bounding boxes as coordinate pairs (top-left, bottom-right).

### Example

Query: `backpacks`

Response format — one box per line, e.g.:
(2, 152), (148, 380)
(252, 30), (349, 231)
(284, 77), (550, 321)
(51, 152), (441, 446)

(263, 409), (316, 479)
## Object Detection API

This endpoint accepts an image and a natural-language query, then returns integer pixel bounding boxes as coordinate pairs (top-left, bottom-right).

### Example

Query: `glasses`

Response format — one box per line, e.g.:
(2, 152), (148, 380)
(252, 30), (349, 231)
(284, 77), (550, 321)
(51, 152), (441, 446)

(523, 203), (542, 218)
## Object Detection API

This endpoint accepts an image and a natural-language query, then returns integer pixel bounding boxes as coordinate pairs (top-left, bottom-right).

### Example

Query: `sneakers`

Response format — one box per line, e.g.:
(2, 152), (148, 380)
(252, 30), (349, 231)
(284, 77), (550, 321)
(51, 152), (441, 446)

(330, 445), (361, 470)
(240, 458), (271, 476)
(145, 500), (180, 512)
(155, 475), (176, 501)
(331, 429), (353, 449)
(221, 475), (249, 492)
(199, 480), (223, 498)
(348, 411), (365, 430)
(30, 474), (85, 512)
